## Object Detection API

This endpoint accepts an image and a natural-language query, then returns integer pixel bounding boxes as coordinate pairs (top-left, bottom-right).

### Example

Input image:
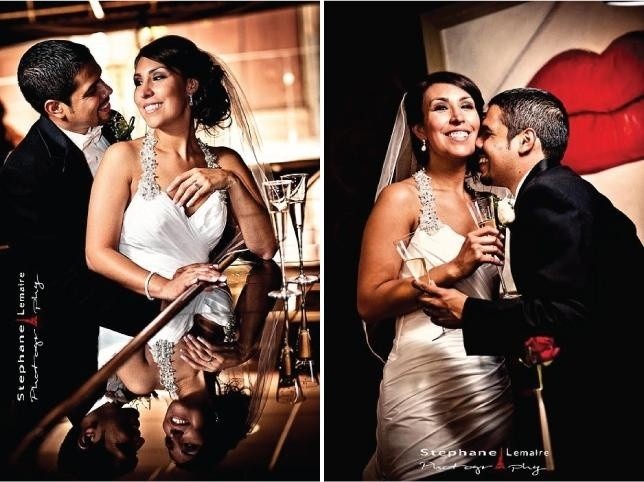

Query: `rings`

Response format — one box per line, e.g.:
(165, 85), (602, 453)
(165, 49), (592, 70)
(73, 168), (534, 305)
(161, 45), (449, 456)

(209, 355), (213, 365)
(195, 182), (200, 190)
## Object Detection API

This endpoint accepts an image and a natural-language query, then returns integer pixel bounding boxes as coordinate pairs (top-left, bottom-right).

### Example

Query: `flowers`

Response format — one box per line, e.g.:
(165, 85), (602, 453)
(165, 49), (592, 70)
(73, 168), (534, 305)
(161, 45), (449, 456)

(519, 333), (562, 370)
(494, 193), (517, 228)
(109, 112), (137, 142)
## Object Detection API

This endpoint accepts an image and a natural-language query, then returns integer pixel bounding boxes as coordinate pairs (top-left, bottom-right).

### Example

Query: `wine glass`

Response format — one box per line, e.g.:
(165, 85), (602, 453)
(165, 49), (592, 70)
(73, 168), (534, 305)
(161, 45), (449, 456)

(274, 294), (304, 404)
(294, 279), (319, 384)
(391, 231), (456, 342)
(262, 178), (301, 299)
(464, 193), (521, 296)
(279, 171), (319, 285)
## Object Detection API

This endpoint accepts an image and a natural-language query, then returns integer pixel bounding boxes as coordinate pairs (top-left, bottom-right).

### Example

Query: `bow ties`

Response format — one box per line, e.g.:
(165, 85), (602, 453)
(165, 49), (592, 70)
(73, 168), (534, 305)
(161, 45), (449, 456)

(107, 384), (129, 404)
(80, 125), (105, 151)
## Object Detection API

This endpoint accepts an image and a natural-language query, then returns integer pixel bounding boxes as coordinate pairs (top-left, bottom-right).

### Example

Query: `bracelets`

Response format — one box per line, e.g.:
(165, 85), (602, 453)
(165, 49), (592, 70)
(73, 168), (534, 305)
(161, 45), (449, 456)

(145, 267), (155, 303)
